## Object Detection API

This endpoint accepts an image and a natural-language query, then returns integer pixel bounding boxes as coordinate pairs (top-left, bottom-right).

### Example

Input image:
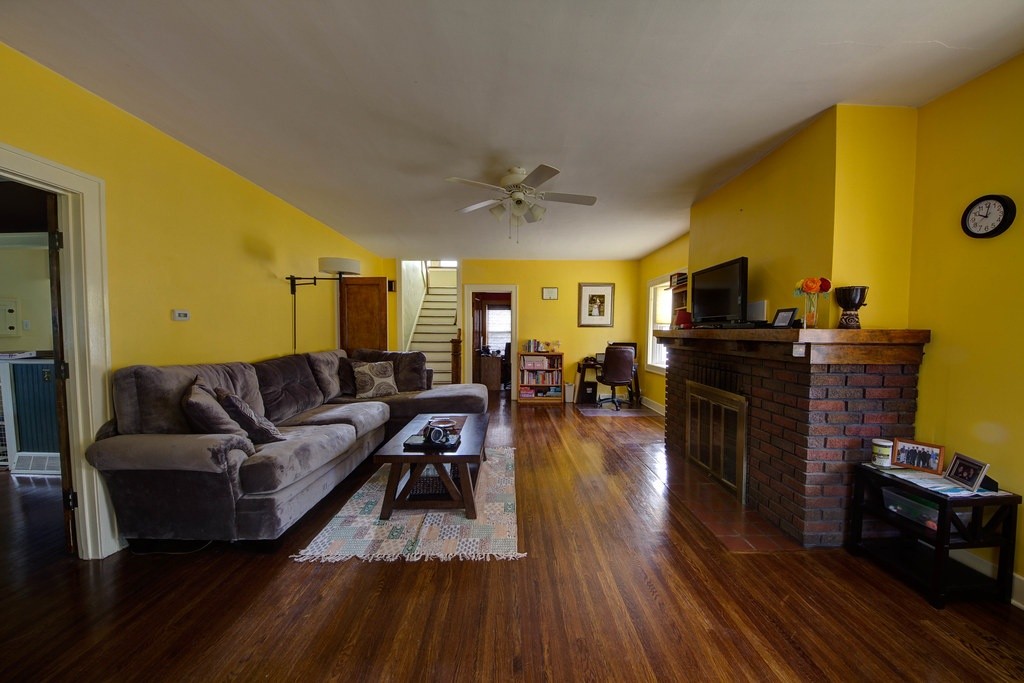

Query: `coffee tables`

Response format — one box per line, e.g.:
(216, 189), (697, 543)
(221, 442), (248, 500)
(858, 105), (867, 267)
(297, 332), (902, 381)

(373, 412), (489, 521)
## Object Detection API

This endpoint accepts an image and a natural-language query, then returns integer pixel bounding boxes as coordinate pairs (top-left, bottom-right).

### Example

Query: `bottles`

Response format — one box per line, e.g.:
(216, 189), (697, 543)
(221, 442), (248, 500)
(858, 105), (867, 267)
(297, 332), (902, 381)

(872, 438), (893, 470)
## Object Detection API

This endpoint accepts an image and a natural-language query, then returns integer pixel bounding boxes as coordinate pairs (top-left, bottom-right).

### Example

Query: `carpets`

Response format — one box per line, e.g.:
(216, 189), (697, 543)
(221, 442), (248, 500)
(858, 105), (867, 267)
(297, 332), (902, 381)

(288, 447), (528, 563)
(575, 402), (660, 416)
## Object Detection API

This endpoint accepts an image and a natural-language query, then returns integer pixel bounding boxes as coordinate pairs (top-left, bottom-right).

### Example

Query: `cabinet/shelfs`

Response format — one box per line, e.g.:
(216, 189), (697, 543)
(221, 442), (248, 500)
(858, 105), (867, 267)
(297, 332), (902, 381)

(516, 351), (565, 408)
(479, 356), (500, 390)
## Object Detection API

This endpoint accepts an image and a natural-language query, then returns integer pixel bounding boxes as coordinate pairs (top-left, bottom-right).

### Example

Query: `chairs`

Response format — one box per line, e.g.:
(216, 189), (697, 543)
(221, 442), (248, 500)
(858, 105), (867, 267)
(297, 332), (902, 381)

(594, 345), (635, 411)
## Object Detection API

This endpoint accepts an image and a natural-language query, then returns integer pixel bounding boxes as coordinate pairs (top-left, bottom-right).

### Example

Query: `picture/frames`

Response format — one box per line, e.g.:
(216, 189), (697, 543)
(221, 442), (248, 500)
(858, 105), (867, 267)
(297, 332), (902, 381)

(892, 437), (944, 475)
(577, 282), (615, 327)
(941, 451), (991, 493)
(769, 309), (797, 329)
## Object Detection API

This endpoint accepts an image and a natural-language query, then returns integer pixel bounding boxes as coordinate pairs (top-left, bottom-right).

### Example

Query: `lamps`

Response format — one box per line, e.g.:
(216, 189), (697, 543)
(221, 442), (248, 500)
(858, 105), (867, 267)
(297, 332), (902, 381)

(285, 257), (361, 354)
(489, 190), (546, 245)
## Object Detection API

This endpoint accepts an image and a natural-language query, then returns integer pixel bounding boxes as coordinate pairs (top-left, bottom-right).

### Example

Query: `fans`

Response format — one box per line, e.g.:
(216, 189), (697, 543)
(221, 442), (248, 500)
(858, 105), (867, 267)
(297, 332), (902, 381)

(445, 163), (598, 213)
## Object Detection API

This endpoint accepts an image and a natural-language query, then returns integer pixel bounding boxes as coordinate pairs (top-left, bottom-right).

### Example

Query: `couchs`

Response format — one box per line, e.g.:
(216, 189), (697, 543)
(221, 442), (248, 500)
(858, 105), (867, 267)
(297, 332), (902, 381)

(85, 348), (489, 553)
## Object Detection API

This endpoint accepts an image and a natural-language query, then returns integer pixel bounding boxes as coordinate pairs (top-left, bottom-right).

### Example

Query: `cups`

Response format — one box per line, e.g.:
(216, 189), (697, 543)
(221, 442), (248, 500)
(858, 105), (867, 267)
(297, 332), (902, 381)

(528, 340), (537, 352)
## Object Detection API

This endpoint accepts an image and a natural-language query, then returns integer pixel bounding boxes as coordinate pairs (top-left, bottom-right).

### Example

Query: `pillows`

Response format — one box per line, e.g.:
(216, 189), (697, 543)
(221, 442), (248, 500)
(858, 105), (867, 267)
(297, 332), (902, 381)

(350, 361), (401, 399)
(182, 372), (256, 453)
(214, 385), (286, 444)
(338, 357), (356, 395)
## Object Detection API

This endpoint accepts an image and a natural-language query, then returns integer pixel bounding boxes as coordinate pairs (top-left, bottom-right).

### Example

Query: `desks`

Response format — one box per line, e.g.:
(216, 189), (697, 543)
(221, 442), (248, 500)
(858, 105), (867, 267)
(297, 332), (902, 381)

(844, 462), (1022, 606)
(578, 361), (640, 407)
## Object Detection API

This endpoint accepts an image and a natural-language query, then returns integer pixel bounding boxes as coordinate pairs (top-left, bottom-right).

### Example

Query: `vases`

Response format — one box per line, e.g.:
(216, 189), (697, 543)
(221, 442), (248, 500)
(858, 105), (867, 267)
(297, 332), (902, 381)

(805, 292), (818, 328)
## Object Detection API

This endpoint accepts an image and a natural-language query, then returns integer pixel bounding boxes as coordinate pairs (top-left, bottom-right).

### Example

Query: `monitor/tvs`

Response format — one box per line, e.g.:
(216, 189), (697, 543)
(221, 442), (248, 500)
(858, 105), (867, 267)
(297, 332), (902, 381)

(691, 256), (747, 324)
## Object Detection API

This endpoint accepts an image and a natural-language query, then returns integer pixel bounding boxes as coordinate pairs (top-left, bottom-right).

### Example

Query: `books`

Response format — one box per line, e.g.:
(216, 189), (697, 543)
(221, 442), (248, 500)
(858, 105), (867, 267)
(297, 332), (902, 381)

(520, 356), (562, 397)
(526, 339), (541, 352)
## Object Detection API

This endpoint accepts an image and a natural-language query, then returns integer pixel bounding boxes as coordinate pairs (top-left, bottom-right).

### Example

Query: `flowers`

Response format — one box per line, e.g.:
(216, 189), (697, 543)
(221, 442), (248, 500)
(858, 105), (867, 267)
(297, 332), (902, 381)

(793, 276), (831, 314)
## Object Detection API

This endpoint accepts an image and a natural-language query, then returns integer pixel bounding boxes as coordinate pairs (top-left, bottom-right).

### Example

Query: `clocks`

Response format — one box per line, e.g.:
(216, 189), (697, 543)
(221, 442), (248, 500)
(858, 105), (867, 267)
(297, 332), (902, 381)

(960, 194), (1017, 238)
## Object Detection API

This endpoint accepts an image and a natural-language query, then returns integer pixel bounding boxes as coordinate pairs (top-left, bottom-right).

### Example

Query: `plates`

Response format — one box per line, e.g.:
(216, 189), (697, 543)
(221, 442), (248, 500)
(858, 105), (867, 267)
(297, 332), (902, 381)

(430, 419), (457, 427)
(537, 350), (549, 353)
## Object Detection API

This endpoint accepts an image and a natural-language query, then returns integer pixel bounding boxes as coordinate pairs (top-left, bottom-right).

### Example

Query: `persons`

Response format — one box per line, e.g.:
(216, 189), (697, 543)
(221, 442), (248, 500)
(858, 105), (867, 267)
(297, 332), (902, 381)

(900, 445), (936, 468)
(954, 465), (976, 481)
